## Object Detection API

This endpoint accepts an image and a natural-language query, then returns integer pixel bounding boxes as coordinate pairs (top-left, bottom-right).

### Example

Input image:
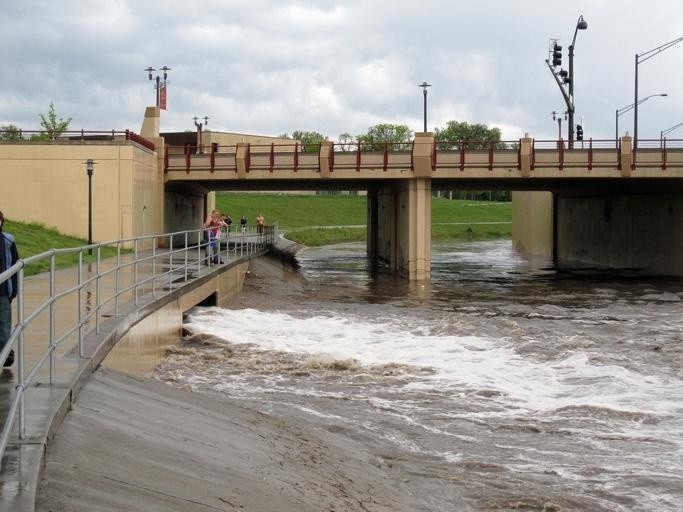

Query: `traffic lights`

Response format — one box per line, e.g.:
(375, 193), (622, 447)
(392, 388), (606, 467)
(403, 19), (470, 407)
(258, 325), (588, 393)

(551, 43), (562, 66)
(575, 125), (583, 142)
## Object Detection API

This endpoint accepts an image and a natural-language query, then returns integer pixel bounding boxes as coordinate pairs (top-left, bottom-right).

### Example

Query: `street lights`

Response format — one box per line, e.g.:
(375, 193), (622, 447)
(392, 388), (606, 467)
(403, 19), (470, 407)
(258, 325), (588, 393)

(566, 15), (587, 151)
(419, 80), (434, 132)
(615, 93), (667, 151)
(80, 159), (99, 256)
(144, 65), (171, 107)
(192, 114), (209, 155)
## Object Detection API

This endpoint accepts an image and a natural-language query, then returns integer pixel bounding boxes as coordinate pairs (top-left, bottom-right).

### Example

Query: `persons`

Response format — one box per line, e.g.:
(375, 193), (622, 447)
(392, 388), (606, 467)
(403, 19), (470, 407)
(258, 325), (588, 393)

(256, 212), (265, 241)
(0, 210), (21, 368)
(203, 209), (232, 266)
(239, 215), (248, 234)
